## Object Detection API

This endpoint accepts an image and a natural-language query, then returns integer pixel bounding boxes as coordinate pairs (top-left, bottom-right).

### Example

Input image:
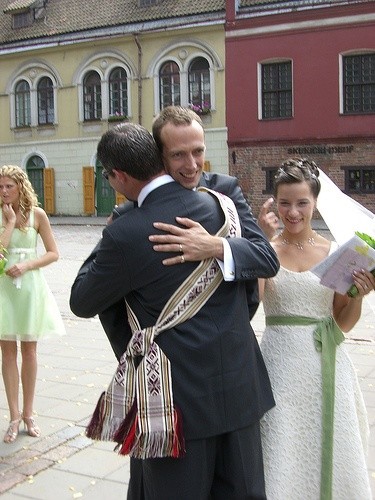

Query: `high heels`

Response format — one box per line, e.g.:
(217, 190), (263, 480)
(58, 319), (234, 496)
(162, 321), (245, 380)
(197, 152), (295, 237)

(3, 413), (23, 444)
(21, 412), (40, 438)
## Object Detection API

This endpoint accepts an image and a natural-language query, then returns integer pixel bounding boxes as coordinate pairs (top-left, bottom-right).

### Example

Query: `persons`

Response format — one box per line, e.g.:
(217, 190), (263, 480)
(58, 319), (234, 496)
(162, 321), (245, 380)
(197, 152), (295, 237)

(98, 106), (280, 500)
(257, 157), (375, 500)
(69, 123), (276, 500)
(0, 165), (67, 444)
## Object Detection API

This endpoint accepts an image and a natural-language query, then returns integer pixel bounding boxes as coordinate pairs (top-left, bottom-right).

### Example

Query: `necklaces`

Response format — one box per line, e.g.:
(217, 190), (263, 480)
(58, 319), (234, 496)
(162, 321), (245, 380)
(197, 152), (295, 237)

(279, 230), (317, 250)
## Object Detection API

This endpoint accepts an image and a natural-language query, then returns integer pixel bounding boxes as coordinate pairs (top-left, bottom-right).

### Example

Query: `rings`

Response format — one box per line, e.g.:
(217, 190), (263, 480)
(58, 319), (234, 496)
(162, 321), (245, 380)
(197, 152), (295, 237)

(179, 243), (183, 251)
(181, 255), (184, 262)
(364, 286), (368, 290)
